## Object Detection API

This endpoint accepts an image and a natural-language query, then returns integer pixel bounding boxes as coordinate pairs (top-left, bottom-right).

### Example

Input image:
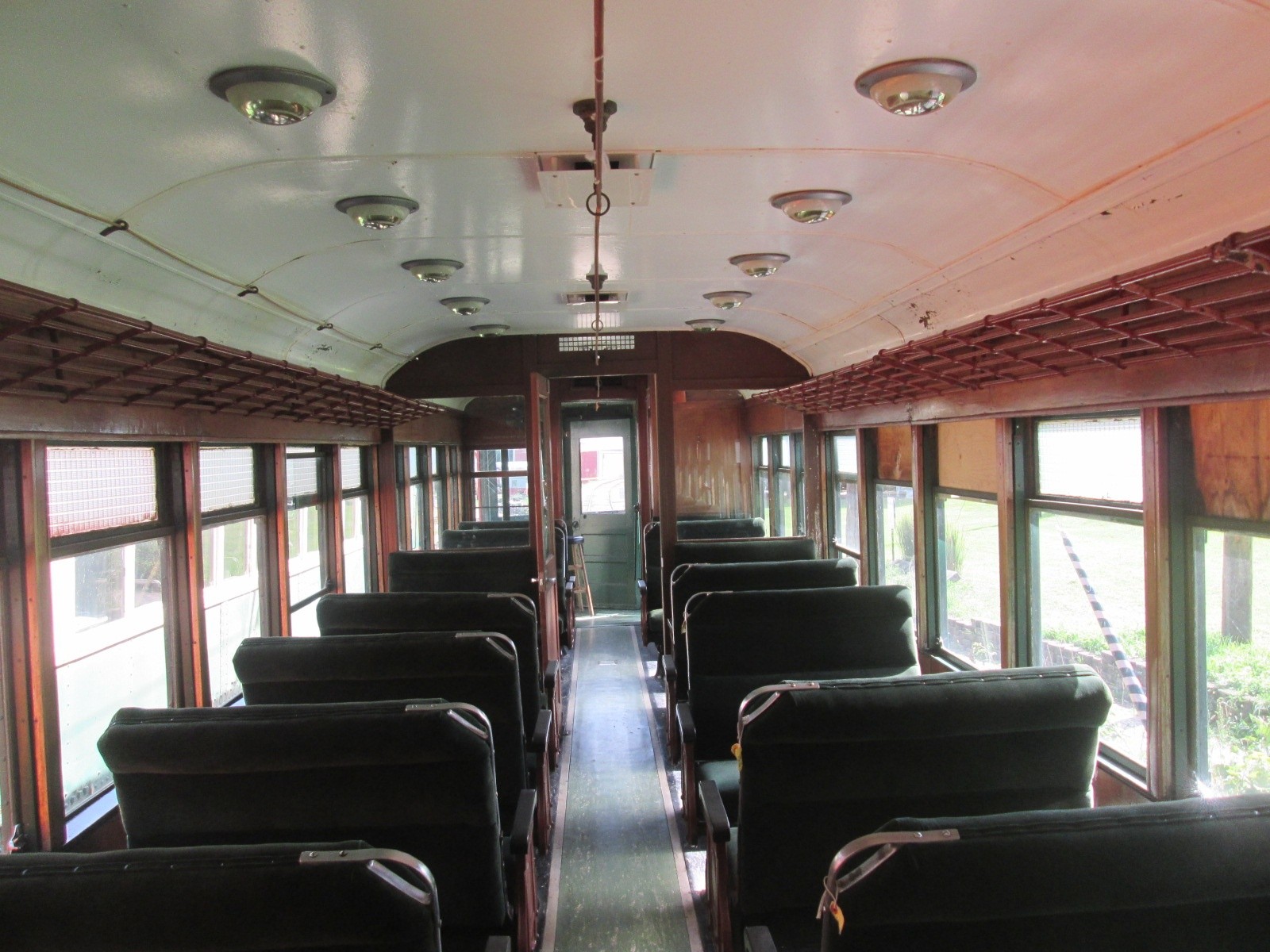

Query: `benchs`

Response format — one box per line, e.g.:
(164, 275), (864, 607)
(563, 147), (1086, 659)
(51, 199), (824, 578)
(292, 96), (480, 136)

(2, 506), (1269, 951)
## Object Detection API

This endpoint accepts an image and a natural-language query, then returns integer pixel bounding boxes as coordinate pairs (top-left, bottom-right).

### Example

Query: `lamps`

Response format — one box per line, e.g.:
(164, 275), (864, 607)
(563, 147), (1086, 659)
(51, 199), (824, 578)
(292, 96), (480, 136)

(855, 57), (976, 118)
(772, 191), (851, 224)
(731, 253), (790, 279)
(207, 67), (339, 129)
(440, 295), (489, 316)
(685, 319), (725, 333)
(704, 291), (752, 310)
(335, 194), (419, 232)
(469, 323), (510, 338)
(400, 257), (465, 283)
